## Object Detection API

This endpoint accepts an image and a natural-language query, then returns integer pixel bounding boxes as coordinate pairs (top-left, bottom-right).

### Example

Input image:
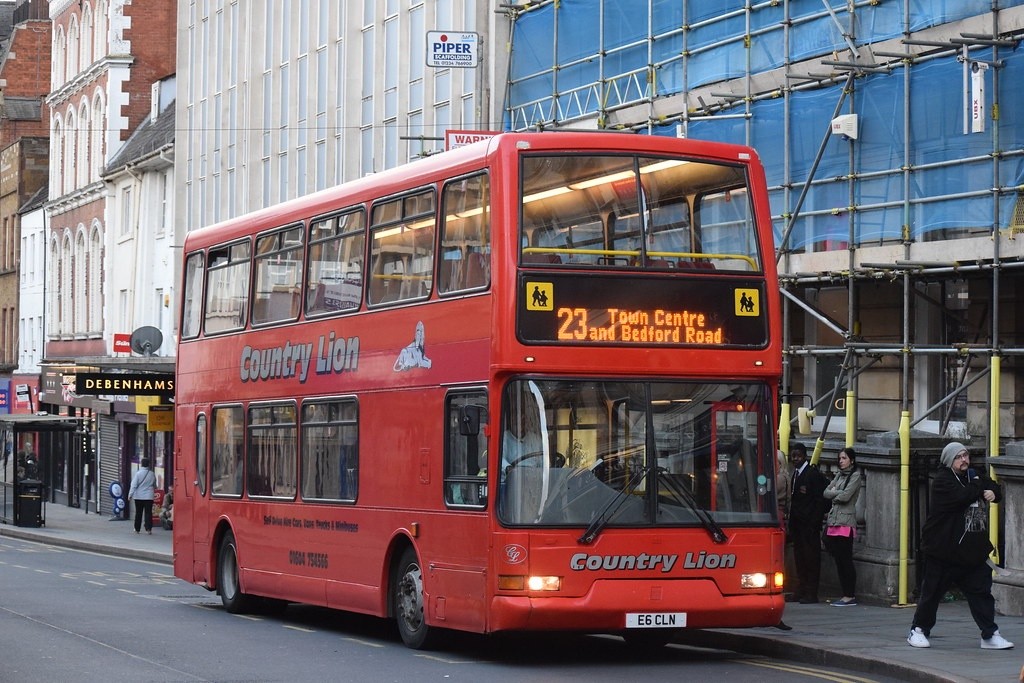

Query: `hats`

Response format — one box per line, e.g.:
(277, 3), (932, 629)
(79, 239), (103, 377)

(940, 442), (967, 469)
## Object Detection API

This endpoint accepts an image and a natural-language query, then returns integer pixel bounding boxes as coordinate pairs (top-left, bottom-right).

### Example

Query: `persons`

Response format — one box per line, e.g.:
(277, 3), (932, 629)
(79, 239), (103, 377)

(721, 438), (791, 523)
(823, 448), (862, 606)
(785, 443), (832, 604)
(907, 442), (1014, 650)
(500, 406), (551, 499)
(161, 484), (174, 530)
(128, 458), (157, 535)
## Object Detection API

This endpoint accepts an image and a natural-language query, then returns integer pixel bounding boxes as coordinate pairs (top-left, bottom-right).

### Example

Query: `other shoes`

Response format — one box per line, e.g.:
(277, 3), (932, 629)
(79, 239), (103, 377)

(134, 528), (142, 534)
(800, 598), (818, 604)
(144, 530), (152, 535)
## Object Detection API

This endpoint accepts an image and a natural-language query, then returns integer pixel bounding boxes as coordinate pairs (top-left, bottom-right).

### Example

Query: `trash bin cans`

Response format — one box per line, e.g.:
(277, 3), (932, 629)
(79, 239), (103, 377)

(18, 479), (43, 528)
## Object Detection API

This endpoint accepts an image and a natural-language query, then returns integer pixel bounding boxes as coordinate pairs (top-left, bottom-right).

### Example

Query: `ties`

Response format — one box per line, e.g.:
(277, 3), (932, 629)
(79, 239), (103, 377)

(791, 470), (798, 496)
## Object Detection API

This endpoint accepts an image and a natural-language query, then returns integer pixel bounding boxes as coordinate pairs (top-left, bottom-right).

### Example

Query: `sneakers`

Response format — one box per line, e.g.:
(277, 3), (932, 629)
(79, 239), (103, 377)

(829, 597), (859, 606)
(981, 630), (1014, 649)
(906, 627), (930, 648)
(826, 594), (844, 603)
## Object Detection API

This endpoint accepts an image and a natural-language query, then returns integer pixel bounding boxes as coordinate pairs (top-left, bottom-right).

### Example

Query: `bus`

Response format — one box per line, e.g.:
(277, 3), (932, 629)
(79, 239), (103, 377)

(173, 133), (815, 649)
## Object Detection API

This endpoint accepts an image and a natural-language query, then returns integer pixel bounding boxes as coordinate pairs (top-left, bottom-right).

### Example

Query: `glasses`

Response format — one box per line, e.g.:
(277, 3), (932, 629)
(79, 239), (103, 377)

(954, 452), (968, 460)
